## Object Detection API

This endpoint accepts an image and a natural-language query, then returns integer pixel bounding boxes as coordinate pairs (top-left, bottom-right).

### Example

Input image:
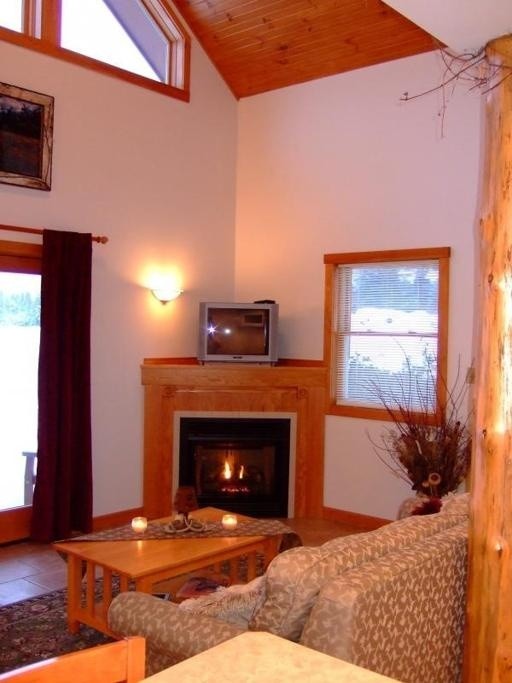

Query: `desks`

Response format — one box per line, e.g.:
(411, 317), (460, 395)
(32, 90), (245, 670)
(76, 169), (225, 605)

(141, 627), (402, 683)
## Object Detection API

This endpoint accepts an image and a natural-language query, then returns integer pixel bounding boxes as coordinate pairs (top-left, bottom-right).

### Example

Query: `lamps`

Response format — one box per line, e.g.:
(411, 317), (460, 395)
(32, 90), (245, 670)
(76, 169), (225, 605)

(153, 284), (184, 304)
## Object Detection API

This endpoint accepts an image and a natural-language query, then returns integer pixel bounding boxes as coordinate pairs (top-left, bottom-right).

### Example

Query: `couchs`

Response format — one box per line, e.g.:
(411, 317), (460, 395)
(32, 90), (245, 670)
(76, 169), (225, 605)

(108, 507), (466, 683)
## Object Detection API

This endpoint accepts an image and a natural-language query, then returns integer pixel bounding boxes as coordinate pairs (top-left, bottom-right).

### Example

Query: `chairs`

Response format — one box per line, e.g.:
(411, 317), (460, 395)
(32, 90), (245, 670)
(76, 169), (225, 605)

(3, 632), (146, 683)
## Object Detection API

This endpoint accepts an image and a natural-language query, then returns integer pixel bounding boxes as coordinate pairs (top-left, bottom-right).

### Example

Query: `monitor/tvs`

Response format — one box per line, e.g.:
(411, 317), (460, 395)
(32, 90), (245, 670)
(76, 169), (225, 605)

(197, 301), (279, 367)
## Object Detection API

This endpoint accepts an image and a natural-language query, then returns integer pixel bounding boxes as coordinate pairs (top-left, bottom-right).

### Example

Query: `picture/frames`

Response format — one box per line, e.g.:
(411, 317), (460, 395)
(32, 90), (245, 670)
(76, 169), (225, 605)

(1, 80), (54, 191)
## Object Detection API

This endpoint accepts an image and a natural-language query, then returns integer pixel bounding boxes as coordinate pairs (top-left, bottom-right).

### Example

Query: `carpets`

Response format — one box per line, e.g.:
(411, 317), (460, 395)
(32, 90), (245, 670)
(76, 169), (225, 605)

(0, 546), (280, 683)
